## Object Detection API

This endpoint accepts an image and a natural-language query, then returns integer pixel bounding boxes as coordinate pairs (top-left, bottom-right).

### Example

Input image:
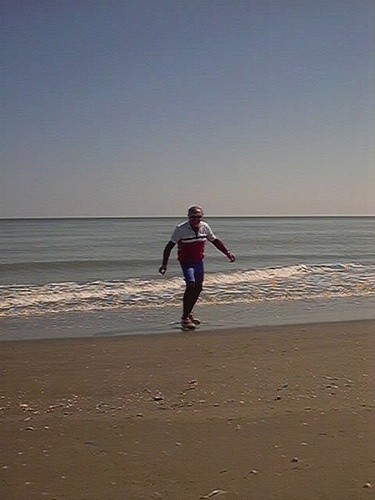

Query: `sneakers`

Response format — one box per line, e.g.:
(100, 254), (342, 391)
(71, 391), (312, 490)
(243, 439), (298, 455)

(180, 318), (197, 328)
(189, 315), (200, 325)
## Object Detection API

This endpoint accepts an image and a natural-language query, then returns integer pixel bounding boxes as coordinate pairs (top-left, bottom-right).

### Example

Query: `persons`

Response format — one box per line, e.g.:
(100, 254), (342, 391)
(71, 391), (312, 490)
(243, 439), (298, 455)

(158, 206), (236, 328)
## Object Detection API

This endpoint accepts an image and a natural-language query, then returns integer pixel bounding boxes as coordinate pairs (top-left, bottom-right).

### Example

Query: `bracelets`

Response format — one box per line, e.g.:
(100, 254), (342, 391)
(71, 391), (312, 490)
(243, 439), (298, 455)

(225, 249), (231, 253)
